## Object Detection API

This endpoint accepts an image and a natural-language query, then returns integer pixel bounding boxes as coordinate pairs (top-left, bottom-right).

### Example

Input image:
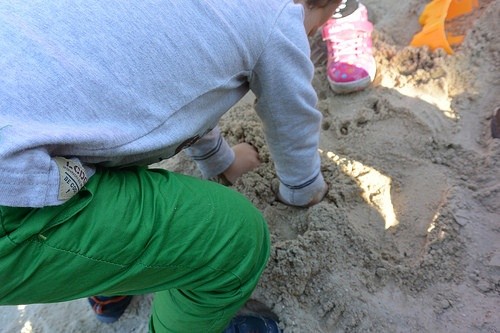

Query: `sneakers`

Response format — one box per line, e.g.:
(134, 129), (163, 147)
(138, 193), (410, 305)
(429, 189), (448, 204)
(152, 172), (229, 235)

(222, 314), (282, 333)
(321, 4), (376, 94)
(89, 295), (131, 323)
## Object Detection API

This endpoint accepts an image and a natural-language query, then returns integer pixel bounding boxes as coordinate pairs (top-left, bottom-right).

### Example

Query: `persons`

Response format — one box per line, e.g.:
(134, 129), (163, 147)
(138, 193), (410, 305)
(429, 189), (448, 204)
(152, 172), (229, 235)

(0, 0), (342, 333)
